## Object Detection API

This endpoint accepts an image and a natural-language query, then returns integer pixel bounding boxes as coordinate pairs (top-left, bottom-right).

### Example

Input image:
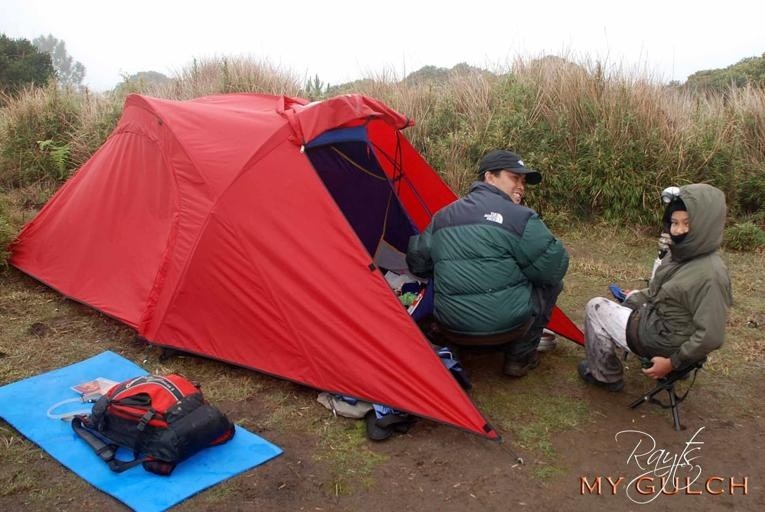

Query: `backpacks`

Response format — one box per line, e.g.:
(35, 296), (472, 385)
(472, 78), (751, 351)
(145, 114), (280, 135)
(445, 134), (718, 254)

(70, 370), (236, 475)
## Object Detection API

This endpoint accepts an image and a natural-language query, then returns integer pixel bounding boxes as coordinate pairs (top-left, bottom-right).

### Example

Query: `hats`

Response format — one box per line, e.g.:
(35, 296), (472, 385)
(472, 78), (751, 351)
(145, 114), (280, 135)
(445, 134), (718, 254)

(478, 150), (542, 185)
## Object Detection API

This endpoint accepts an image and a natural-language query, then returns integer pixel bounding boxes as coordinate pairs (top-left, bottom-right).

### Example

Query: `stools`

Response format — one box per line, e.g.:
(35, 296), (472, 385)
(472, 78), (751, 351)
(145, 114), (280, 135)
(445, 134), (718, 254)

(628, 356), (706, 432)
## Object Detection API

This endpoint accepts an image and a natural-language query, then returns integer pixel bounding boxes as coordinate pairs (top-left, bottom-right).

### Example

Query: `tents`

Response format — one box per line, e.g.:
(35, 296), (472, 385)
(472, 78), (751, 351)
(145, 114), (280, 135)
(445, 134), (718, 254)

(8, 89), (586, 441)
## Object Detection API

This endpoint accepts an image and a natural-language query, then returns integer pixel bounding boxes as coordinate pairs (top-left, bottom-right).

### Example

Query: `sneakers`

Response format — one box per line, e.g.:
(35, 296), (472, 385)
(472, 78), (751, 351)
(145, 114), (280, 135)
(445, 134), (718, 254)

(576, 358), (625, 392)
(502, 353), (540, 379)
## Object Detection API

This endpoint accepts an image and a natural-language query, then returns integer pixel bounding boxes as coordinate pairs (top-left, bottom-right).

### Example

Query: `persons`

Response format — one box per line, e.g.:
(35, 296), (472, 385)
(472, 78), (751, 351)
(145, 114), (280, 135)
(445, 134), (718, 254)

(578, 181), (733, 393)
(403, 146), (572, 383)
